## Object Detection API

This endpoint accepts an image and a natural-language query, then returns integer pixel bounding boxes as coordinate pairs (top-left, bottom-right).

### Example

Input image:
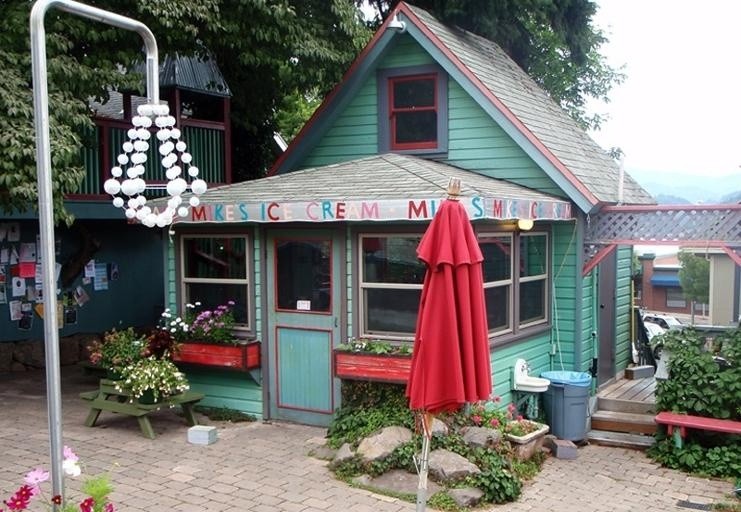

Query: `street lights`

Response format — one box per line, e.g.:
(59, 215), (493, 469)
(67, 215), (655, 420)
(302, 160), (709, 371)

(27, 0), (209, 512)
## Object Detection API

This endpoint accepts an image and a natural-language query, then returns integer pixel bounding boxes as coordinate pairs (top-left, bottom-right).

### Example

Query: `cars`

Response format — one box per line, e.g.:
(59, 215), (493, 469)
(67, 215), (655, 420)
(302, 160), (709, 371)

(642, 312), (684, 361)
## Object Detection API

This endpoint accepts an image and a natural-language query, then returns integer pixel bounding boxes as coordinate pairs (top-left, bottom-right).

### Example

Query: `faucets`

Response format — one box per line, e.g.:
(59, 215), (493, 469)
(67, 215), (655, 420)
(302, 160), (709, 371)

(522, 362), (531, 373)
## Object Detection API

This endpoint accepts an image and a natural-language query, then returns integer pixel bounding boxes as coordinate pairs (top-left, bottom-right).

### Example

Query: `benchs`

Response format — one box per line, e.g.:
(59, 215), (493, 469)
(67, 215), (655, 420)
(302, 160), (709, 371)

(79, 389), (102, 428)
(136, 391), (205, 439)
(654, 412), (741, 448)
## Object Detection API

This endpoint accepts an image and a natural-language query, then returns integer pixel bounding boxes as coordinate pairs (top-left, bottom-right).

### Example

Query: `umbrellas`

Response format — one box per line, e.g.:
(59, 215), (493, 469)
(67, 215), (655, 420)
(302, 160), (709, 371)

(402, 176), (495, 511)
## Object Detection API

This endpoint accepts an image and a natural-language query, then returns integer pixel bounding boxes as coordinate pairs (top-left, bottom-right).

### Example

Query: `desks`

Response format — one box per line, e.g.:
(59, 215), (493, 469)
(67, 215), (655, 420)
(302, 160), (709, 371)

(85, 378), (199, 440)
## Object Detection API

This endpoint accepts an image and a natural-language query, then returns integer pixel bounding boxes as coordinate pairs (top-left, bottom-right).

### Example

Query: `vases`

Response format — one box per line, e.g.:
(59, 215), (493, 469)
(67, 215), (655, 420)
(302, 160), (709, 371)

(168, 340), (261, 372)
(496, 417), (552, 465)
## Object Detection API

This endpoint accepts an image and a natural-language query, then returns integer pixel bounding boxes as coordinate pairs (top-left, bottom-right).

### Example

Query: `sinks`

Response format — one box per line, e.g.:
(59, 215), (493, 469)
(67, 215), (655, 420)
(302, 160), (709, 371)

(516, 377), (551, 392)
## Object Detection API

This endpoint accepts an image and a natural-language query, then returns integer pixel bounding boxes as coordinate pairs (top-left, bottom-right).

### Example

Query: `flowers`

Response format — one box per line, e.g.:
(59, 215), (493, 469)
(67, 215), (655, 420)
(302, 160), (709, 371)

(86, 326), (191, 412)
(156, 300), (242, 344)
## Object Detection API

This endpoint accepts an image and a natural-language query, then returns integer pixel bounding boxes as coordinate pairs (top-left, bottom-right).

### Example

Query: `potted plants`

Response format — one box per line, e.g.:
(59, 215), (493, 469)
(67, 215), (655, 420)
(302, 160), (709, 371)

(332, 336), (412, 385)
